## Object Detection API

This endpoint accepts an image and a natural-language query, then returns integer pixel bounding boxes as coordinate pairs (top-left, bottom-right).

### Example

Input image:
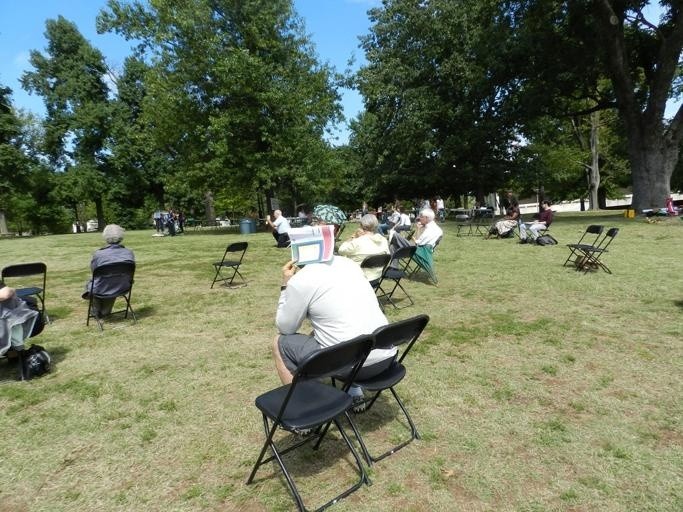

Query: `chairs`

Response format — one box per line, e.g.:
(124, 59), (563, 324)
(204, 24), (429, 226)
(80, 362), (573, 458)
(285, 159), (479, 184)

(313, 312), (431, 471)
(246, 334), (378, 512)
(1, 262), (50, 325)
(82, 260), (136, 331)
(210, 242), (247, 288)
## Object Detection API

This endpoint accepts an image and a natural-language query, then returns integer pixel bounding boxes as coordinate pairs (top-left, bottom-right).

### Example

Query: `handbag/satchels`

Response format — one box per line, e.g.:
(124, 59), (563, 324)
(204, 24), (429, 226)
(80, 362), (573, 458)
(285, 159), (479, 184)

(21, 343), (51, 379)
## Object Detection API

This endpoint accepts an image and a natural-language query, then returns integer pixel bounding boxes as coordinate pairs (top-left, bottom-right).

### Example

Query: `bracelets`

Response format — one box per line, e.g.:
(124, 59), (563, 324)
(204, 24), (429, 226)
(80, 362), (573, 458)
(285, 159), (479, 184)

(280, 286), (287, 291)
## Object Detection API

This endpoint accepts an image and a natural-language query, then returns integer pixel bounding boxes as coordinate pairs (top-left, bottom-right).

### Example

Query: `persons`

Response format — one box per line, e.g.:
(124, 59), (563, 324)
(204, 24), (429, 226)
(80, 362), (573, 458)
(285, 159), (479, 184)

(519, 200), (552, 244)
(504, 192), (518, 220)
(153, 206), (184, 237)
(86, 224), (134, 317)
(485, 201), (520, 239)
(265, 196), (445, 288)
(272, 255), (398, 435)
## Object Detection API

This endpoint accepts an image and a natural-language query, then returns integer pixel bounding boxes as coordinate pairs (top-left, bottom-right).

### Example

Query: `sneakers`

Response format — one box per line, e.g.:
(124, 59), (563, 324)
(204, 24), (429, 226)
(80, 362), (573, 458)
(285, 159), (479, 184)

(347, 393), (366, 413)
